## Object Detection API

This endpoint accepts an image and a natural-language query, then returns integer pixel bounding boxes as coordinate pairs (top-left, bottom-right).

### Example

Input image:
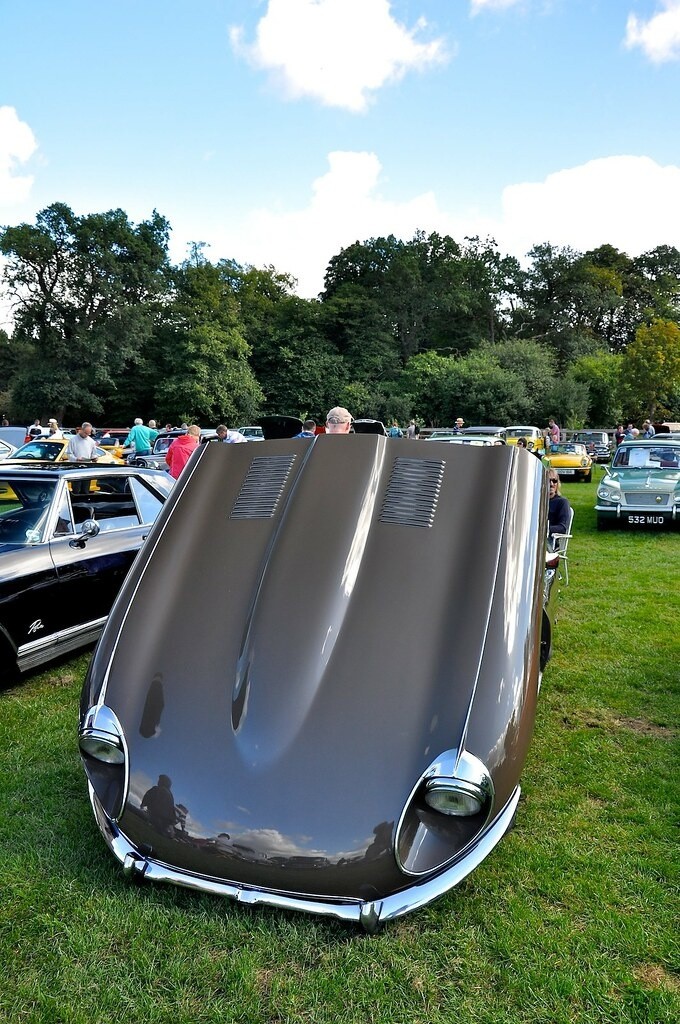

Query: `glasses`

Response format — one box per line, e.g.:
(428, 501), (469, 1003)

(517, 443), (524, 445)
(549, 478), (558, 483)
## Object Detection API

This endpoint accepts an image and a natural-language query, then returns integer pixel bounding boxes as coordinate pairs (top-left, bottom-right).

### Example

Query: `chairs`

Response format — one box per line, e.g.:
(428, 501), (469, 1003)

(72, 503), (94, 524)
(551, 508), (575, 586)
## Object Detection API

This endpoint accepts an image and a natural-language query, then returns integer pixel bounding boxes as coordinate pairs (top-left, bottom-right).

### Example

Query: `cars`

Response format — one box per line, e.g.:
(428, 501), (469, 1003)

(505, 426), (544, 452)
(0, 425), (263, 464)
(541, 441), (593, 484)
(0, 464), (177, 687)
(75, 423), (557, 925)
(595, 433), (680, 530)
(570, 431), (612, 463)
(424, 426), (506, 447)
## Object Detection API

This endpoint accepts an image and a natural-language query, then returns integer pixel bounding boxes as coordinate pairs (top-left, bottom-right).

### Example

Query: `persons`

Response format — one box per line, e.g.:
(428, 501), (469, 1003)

(545, 468), (572, 607)
(291, 419), (316, 439)
(215, 424), (248, 443)
(165, 424), (202, 480)
(119, 418), (188, 456)
(326, 406), (355, 434)
(26, 418), (98, 463)
(615, 419), (655, 450)
(2, 413), (9, 426)
(389, 422), (404, 438)
(406, 418), (419, 440)
(516, 415), (560, 462)
(139, 775), (233, 857)
(453, 417), (466, 435)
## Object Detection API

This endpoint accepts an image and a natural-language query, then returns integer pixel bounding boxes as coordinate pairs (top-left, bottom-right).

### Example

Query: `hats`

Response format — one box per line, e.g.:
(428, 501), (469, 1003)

(327, 406), (352, 423)
(455, 418), (465, 423)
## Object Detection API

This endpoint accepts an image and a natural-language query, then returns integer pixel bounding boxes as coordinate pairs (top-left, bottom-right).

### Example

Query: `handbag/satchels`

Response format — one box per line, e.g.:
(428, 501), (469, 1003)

(415, 425), (420, 435)
